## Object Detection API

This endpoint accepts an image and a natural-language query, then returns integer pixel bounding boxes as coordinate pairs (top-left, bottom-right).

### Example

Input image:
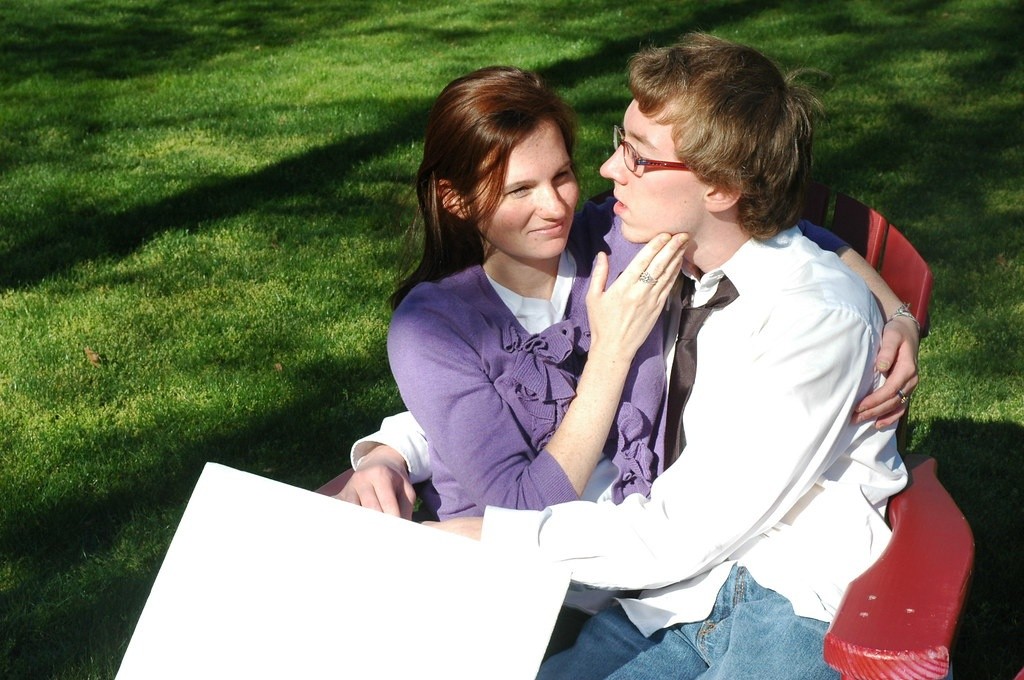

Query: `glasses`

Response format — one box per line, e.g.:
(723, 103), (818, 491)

(614, 124), (695, 178)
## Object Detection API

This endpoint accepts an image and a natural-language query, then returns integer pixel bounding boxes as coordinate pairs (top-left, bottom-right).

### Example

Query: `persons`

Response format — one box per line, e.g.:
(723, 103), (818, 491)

(386, 67), (919, 680)
(330, 31), (908, 680)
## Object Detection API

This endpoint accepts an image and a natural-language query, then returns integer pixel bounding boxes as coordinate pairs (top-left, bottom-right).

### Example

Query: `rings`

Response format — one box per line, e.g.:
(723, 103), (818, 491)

(639, 271), (657, 285)
(898, 389), (908, 404)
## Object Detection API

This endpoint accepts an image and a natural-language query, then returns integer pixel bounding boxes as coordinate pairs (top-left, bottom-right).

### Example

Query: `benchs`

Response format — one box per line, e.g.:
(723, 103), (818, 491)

(798, 166), (974, 679)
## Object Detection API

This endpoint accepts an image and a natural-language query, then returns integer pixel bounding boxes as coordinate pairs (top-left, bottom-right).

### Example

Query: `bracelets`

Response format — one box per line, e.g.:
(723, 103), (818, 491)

(885, 302), (920, 332)
